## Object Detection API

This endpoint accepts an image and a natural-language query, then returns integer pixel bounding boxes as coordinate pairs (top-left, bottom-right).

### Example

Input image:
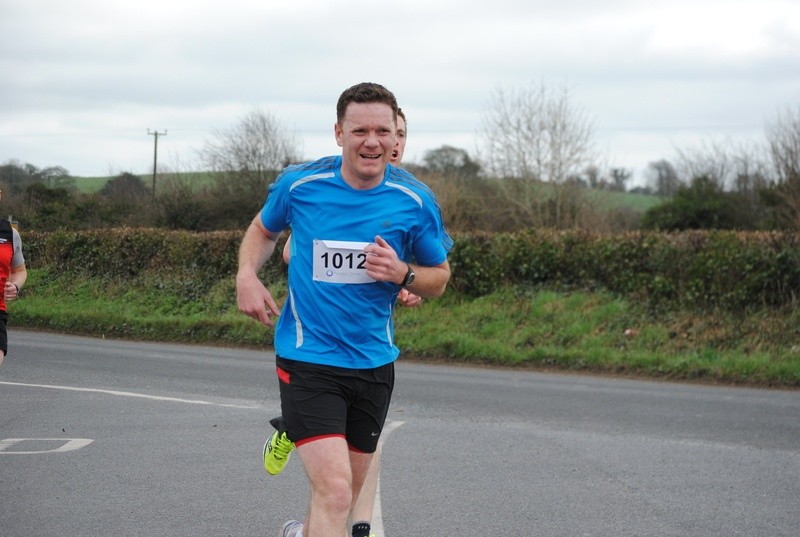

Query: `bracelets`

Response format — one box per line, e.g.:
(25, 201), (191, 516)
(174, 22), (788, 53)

(14, 284), (19, 296)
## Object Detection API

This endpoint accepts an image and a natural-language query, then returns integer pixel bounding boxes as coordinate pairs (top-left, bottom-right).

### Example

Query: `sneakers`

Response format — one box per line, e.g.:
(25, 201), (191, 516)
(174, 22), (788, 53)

(263, 429), (295, 475)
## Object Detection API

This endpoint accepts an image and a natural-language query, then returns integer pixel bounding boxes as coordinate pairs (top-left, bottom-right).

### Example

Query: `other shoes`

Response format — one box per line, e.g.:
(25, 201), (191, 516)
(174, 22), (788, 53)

(279, 520), (303, 537)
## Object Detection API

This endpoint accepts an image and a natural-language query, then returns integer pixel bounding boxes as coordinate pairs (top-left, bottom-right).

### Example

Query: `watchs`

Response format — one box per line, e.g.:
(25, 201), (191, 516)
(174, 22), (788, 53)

(399, 264), (415, 288)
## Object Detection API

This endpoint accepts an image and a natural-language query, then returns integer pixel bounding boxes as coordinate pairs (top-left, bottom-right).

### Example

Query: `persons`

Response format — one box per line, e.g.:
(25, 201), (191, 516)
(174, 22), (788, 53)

(0, 188), (27, 366)
(234, 82), (456, 537)
